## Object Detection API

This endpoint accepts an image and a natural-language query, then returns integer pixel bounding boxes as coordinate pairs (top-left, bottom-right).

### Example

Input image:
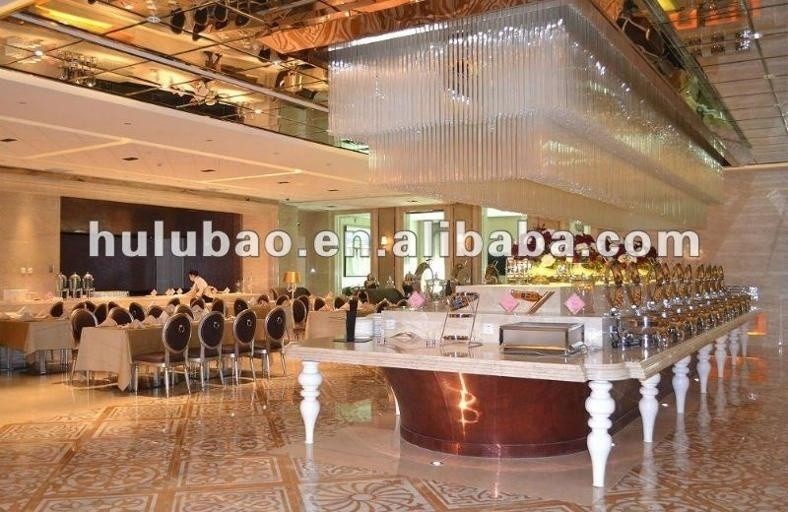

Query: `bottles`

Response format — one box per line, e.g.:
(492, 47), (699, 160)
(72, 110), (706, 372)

(612, 285), (751, 351)
(426, 272), (447, 310)
(453, 256), (473, 284)
(571, 263), (596, 314)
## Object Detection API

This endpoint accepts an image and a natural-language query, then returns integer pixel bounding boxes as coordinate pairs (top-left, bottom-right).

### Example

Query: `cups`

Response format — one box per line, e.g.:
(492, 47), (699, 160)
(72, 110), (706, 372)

(506, 255), (570, 285)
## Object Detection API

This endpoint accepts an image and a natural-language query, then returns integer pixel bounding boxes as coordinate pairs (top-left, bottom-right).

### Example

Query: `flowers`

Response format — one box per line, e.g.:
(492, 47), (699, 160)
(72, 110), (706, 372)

(510, 222), (659, 269)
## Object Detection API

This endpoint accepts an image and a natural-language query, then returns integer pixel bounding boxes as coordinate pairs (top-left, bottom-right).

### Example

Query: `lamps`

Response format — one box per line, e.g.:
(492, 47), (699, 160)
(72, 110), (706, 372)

(303, 1), (737, 236)
(281, 270), (301, 299)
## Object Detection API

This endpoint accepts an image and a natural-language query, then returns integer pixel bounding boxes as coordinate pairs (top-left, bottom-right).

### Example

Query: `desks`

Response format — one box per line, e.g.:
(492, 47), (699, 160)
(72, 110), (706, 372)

(282, 276), (768, 488)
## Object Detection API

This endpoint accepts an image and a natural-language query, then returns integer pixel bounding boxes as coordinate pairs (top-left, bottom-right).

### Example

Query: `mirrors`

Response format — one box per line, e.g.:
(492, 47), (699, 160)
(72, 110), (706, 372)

(0, 2), (368, 155)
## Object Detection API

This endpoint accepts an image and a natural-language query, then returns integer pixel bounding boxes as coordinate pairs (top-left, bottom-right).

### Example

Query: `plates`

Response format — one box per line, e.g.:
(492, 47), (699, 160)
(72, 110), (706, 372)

(353, 313), (383, 338)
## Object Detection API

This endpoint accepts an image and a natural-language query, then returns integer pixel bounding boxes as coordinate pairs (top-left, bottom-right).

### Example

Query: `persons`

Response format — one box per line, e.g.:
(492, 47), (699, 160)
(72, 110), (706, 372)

(190, 50), (224, 105)
(386, 285), (425, 308)
(183, 270), (209, 297)
(338, 290), (385, 312)
(400, 272), (414, 296)
(363, 272), (379, 291)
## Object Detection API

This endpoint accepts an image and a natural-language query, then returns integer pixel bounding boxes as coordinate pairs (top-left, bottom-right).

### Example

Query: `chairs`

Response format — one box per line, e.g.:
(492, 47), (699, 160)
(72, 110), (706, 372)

(1, 286), (415, 399)
(56, 49), (100, 89)
(165, 1), (273, 45)
